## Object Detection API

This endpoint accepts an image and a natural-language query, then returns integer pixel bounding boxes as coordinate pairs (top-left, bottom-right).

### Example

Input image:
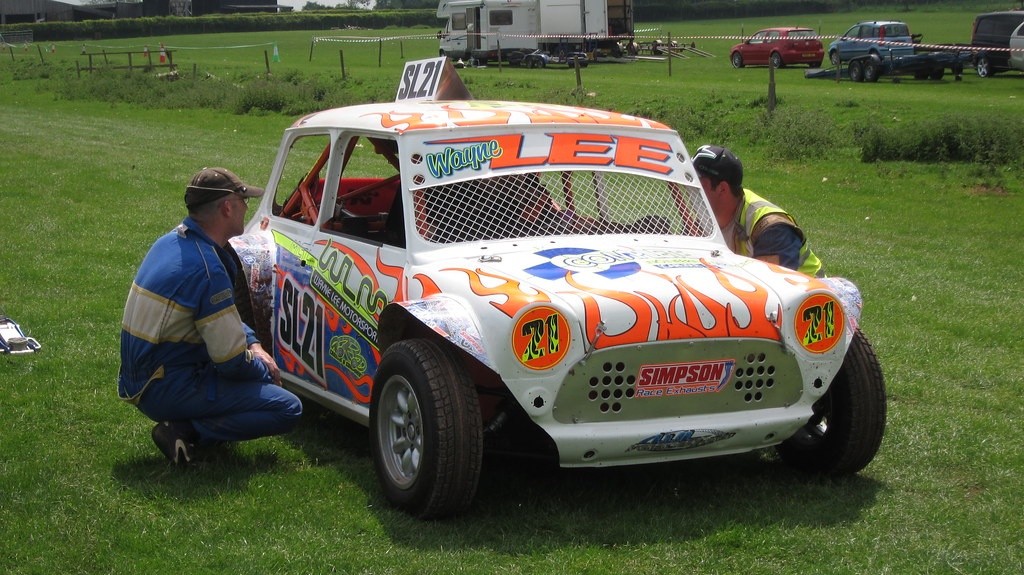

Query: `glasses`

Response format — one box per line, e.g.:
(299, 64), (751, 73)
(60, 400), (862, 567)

(215, 196), (250, 209)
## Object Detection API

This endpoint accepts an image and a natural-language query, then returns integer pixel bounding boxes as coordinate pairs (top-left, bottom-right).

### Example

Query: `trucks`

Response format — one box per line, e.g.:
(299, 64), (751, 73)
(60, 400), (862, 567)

(435, 0), (636, 66)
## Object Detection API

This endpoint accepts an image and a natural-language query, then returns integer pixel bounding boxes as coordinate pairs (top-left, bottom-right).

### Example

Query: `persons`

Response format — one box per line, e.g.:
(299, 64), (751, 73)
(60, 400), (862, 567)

(692, 143), (830, 277)
(119, 167), (303, 467)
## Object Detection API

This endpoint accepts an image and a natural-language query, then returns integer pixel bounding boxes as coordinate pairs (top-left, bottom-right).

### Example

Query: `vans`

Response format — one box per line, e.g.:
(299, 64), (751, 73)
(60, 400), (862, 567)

(971, 12), (1024, 78)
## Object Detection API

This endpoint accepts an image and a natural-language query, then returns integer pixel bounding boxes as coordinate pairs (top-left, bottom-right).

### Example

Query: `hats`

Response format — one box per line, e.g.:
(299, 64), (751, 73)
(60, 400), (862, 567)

(691, 145), (743, 184)
(184, 167), (264, 198)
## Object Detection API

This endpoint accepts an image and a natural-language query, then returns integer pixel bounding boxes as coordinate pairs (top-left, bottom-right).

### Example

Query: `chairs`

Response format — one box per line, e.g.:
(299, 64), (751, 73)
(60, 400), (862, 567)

(381, 163), (484, 233)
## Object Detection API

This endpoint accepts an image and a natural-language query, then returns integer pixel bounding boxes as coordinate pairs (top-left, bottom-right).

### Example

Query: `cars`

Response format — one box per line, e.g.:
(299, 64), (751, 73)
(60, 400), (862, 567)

(729, 26), (826, 69)
(828, 20), (915, 65)
(227, 99), (888, 523)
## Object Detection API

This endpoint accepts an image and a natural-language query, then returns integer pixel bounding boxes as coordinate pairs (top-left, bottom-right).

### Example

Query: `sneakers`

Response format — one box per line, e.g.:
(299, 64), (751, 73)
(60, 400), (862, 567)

(152, 421), (196, 469)
